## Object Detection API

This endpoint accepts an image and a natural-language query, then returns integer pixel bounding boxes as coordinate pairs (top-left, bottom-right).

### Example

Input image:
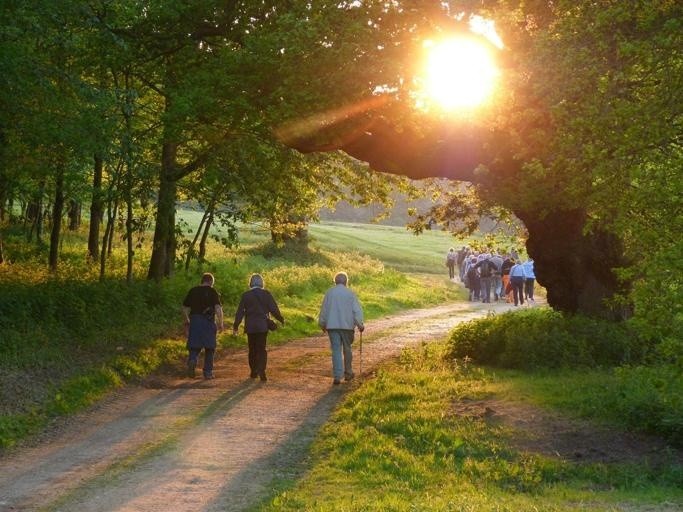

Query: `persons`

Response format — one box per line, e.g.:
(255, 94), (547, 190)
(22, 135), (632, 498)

(444, 242), (537, 307)
(318, 271), (365, 385)
(181, 272), (224, 380)
(232, 272), (284, 381)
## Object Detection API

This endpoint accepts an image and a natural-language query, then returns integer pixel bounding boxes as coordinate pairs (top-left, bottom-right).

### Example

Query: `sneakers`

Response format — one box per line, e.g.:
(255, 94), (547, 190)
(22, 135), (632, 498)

(251, 373), (257, 377)
(261, 375), (267, 381)
(334, 376), (339, 384)
(344, 372), (352, 380)
(189, 360), (196, 379)
(206, 376), (214, 379)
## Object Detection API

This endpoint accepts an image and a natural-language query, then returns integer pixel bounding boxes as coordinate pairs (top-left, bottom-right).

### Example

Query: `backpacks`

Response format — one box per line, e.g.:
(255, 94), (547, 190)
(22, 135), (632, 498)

(467, 265), (478, 279)
(481, 260), (491, 277)
(503, 262), (512, 274)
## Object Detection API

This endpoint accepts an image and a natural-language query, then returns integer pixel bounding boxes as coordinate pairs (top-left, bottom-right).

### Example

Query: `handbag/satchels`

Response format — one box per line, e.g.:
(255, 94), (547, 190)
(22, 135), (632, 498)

(506, 284), (513, 294)
(268, 320), (277, 331)
